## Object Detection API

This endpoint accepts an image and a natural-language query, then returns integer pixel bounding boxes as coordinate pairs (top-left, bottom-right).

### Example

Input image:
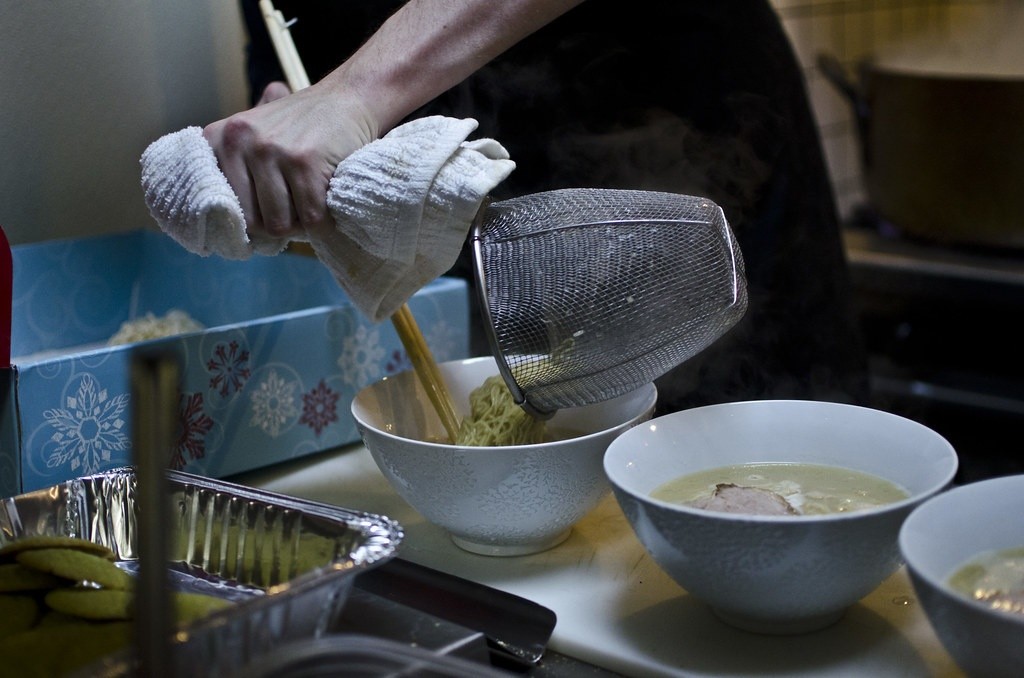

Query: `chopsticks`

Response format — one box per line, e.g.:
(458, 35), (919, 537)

(260, 0), (464, 446)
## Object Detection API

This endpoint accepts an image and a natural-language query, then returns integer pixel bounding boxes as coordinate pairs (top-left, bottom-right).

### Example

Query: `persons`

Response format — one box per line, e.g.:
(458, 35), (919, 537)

(199, 0), (871, 420)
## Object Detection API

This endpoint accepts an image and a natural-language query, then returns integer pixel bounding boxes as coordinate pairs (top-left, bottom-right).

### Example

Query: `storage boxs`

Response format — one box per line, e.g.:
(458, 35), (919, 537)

(0, 464), (407, 678)
(7, 230), (469, 495)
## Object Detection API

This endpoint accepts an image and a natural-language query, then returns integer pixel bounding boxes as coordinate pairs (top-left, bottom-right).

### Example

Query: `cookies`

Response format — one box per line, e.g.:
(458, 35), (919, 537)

(0, 536), (242, 678)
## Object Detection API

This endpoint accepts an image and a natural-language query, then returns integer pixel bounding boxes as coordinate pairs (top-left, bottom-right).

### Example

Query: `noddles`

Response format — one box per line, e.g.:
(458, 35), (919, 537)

(649, 463), (911, 517)
(409, 356), (593, 446)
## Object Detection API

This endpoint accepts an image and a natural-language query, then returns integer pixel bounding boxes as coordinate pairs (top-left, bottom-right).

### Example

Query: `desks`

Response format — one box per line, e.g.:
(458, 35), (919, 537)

(244, 441), (968, 678)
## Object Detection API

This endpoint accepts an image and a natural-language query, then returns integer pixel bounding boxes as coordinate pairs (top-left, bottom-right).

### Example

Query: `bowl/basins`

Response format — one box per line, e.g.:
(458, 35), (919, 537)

(895, 473), (1024, 678)
(603, 399), (960, 634)
(350, 355), (658, 558)
